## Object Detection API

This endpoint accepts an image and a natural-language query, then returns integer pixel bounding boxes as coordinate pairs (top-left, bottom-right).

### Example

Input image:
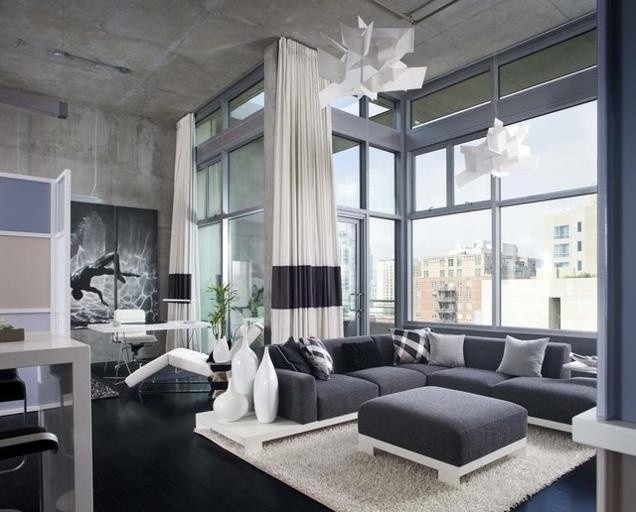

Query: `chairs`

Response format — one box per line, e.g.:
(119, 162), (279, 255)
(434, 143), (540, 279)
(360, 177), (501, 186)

(111, 308), (158, 372)
(0, 368), (59, 478)
(124, 321), (265, 404)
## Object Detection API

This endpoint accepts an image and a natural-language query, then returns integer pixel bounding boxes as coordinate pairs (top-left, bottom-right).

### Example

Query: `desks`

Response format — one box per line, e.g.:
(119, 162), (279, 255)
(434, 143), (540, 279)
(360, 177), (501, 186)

(0, 334), (94, 511)
(87, 319), (213, 388)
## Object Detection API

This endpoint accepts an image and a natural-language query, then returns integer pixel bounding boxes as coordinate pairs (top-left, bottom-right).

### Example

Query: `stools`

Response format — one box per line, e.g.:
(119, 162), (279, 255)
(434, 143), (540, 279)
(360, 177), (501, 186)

(354, 383), (531, 487)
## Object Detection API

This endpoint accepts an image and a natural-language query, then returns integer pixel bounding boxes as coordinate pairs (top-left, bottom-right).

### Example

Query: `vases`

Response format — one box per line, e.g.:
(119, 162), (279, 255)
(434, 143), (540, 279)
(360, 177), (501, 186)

(213, 322), (279, 424)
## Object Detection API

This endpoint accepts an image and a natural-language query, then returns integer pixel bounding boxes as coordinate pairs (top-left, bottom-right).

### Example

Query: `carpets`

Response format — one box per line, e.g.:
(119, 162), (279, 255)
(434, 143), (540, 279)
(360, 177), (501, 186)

(91, 369), (121, 402)
(192, 418), (598, 512)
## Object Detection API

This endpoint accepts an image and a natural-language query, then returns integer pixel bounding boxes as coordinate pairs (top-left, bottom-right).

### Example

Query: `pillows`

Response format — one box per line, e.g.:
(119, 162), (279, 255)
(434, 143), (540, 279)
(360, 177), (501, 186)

(275, 328), (550, 380)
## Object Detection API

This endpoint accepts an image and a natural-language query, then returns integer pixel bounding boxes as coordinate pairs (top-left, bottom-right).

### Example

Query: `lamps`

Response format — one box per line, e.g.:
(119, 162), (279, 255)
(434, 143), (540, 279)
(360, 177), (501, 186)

(311, 0), (428, 109)
(454, 1), (534, 188)
(162, 272), (195, 349)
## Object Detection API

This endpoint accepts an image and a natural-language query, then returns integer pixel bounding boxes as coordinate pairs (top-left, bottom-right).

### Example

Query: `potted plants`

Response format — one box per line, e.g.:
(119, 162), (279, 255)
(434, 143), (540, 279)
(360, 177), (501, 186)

(205, 283), (239, 363)
(0, 317), (27, 345)
